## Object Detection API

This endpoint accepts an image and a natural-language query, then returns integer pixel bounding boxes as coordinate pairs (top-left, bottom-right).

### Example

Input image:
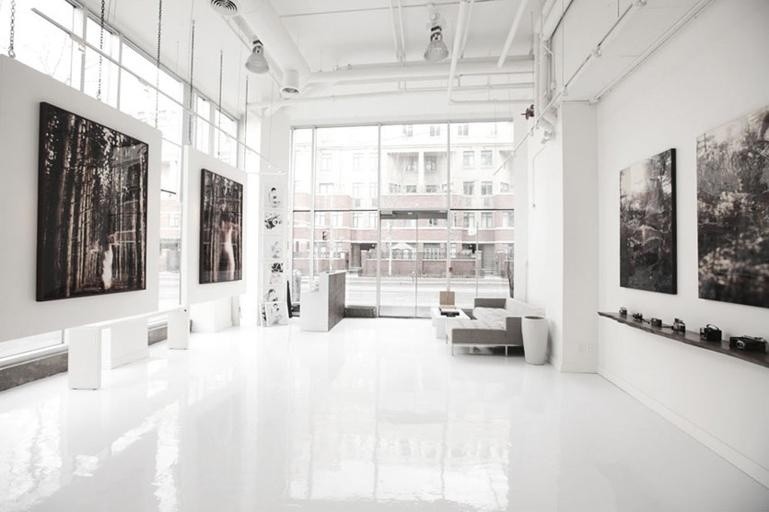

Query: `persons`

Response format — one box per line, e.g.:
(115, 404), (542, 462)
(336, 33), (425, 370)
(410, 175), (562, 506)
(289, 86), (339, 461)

(203, 200), (228, 281)
(220, 212), (241, 281)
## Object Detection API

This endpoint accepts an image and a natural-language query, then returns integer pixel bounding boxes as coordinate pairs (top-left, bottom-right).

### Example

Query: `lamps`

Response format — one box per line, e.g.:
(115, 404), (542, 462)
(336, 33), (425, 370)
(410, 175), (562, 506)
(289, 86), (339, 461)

(209, 1), (450, 96)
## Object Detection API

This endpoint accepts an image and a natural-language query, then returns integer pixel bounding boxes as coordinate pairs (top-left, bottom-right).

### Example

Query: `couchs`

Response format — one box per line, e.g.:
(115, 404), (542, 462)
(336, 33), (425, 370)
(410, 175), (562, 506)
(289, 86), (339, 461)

(449, 298), (547, 357)
